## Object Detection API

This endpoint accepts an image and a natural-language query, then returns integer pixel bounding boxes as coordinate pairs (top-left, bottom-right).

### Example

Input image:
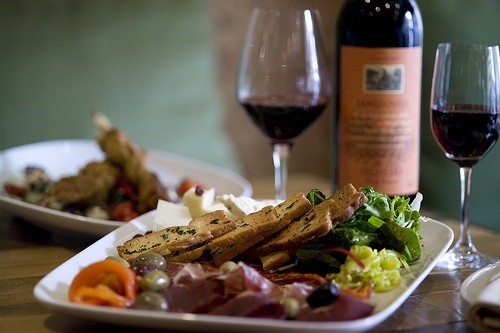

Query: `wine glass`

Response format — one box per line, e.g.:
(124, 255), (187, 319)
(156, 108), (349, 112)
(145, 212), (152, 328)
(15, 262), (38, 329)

(430, 41), (495, 271)
(236, 7), (331, 202)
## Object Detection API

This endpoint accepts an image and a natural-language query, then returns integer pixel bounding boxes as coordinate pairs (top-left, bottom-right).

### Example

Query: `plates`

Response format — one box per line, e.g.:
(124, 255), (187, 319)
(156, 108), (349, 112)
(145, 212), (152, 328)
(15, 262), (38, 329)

(33, 197), (454, 333)
(1, 137), (253, 232)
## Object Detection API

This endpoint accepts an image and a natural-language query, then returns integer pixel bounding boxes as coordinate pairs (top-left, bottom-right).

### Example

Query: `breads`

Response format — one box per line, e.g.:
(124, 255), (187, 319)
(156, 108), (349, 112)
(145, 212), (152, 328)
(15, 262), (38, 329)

(114, 183), (369, 272)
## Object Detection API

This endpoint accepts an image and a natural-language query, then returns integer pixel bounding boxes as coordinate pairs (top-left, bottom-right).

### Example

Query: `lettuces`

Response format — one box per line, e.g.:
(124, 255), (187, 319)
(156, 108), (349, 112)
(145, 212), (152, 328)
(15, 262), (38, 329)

(287, 186), (423, 277)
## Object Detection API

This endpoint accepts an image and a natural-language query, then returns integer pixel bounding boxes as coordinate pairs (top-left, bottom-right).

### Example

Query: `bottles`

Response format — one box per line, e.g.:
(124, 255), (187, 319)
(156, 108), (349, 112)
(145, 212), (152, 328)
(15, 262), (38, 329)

(335, 1), (424, 197)
